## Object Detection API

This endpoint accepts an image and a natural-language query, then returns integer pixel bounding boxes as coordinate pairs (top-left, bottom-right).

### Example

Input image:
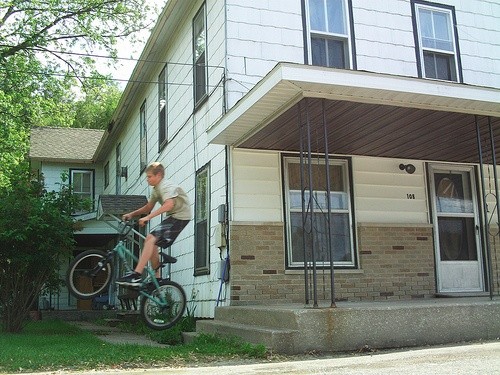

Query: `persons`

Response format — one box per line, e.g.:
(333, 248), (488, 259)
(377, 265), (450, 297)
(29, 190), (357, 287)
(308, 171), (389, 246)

(113, 162), (192, 289)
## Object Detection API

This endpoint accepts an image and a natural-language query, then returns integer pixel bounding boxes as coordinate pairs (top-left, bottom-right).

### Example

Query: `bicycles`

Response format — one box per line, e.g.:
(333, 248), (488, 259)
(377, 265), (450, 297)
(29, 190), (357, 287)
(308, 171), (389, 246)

(68, 209), (186, 330)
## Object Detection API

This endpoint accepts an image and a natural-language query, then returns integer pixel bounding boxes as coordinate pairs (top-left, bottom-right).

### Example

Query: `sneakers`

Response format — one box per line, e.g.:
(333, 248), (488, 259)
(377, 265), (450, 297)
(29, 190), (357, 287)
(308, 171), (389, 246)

(114, 271), (144, 287)
